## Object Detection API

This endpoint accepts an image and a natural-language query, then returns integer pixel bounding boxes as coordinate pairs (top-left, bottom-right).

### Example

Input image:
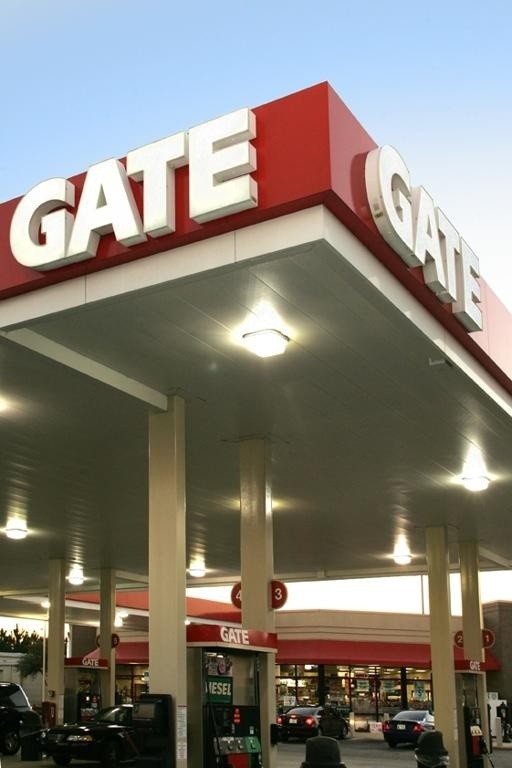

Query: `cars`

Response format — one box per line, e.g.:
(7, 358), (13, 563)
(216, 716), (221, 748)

(276, 704), (348, 741)
(0, 682), (48, 756)
(32, 703), (133, 767)
(382, 709), (434, 749)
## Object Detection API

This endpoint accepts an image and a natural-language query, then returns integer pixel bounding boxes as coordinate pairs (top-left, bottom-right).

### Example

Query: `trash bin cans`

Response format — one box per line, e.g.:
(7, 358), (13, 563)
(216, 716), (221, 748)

(42, 701), (55, 728)
(132, 694), (176, 768)
